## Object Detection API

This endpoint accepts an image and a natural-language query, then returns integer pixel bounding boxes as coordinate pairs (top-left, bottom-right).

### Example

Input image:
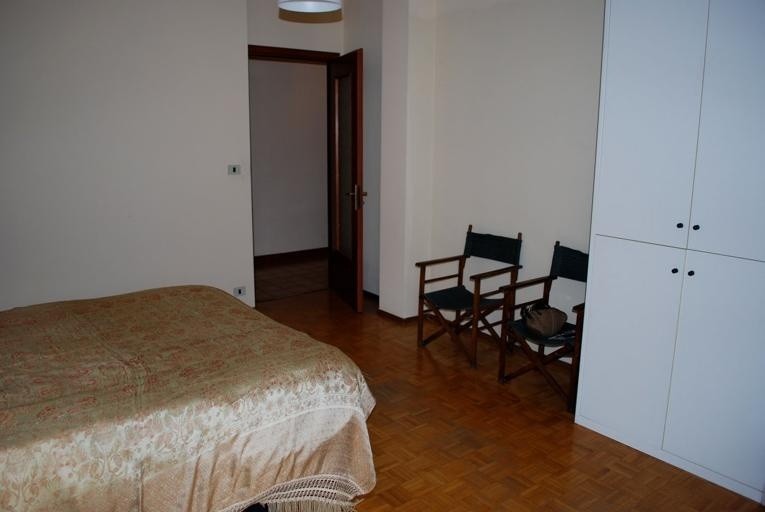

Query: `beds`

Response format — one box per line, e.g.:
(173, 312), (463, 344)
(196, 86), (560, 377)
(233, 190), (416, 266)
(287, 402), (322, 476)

(0, 281), (377, 512)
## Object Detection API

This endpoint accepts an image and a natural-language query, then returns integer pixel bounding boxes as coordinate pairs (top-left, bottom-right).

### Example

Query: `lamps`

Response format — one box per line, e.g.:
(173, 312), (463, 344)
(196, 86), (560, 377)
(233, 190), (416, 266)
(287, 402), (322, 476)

(276, 1), (343, 14)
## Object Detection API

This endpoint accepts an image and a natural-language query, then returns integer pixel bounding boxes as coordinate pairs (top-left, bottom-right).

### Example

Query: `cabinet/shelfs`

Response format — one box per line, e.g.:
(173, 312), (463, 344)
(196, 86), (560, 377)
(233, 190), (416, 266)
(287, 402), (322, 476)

(589, 1), (764, 266)
(570, 232), (765, 504)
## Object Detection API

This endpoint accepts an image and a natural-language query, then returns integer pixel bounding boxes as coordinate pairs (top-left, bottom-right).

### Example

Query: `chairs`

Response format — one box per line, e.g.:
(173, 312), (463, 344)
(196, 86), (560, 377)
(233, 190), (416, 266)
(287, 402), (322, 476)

(413, 223), (525, 371)
(496, 239), (588, 415)
